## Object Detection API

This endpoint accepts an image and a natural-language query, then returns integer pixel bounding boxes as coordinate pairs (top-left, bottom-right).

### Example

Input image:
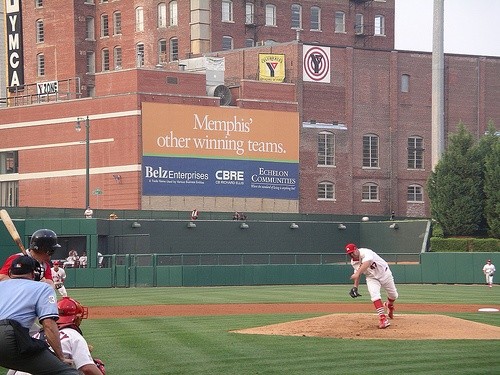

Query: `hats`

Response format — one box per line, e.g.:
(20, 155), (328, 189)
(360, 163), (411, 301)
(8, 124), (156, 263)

(74, 300), (84, 319)
(345, 243), (357, 255)
(53, 260), (60, 265)
(11, 255), (35, 274)
(51, 297), (77, 323)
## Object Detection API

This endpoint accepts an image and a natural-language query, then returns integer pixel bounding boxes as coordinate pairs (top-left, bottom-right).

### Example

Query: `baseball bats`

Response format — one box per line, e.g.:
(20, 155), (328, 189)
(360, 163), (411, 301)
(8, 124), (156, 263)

(0, 209), (28, 256)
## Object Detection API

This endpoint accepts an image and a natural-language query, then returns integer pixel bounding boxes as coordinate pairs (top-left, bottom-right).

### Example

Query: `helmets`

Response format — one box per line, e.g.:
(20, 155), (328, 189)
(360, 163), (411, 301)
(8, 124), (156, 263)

(29, 228), (61, 250)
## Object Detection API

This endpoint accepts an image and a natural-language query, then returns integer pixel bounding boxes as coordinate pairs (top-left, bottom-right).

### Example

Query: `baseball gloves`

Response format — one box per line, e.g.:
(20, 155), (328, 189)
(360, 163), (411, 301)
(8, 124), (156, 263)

(349, 287), (362, 298)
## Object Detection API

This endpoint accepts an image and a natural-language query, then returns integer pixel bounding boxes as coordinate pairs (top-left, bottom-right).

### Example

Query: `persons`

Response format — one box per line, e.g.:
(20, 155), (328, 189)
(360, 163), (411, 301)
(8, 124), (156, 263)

(0, 254), (82, 375)
(190, 208), (200, 219)
(62, 248), (105, 269)
(83, 206), (94, 219)
(482, 257), (496, 288)
(232, 210), (249, 220)
(7, 297), (107, 374)
(0, 228), (61, 375)
(48, 260), (68, 298)
(345, 243), (397, 329)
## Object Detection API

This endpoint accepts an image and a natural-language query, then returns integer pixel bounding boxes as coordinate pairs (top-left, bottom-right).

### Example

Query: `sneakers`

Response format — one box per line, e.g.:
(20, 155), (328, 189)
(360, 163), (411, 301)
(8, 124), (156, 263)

(379, 318), (390, 329)
(383, 302), (395, 319)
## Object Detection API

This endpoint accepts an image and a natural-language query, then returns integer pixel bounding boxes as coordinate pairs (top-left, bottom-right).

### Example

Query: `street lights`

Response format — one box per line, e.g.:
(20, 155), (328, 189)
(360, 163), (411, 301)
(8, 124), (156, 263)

(74, 115), (91, 211)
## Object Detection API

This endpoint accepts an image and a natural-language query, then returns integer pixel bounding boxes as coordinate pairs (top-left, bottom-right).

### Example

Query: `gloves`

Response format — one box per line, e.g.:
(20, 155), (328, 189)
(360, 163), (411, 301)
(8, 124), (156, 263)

(349, 287), (361, 298)
